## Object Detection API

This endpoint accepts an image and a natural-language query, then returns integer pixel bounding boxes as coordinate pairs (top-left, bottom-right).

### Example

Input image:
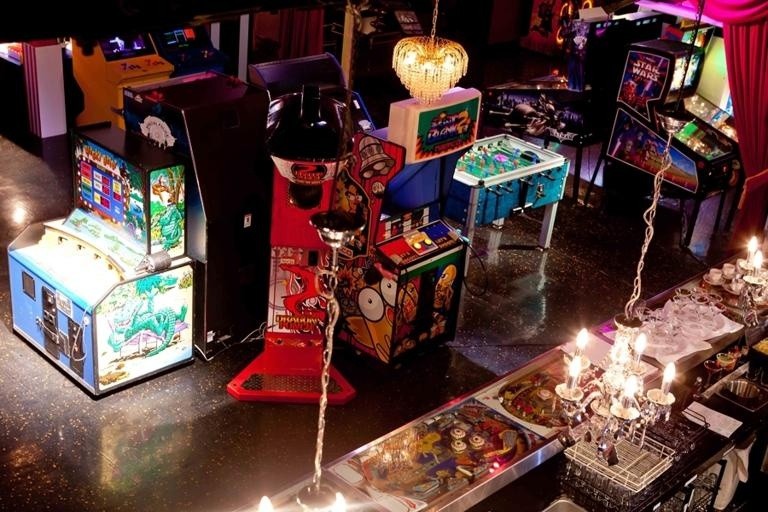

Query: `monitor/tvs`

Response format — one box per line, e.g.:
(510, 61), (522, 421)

(97, 31), (157, 62)
(160, 26), (200, 51)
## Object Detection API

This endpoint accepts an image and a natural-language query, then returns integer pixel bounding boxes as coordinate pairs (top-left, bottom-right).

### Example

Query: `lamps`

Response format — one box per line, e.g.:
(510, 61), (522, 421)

(737, 235), (768, 329)
(257, 1), (369, 512)
(391, 0), (469, 105)
(554, 0), (706, 467)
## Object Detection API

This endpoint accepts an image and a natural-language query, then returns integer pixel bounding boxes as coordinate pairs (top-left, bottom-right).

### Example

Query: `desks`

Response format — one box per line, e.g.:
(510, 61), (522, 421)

(445, 133), (570, 276)
(519, 129), (605, 200)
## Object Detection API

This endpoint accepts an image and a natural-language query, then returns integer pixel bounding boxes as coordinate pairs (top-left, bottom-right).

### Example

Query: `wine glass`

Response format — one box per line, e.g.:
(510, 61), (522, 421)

(646, 287), (727, 357)
